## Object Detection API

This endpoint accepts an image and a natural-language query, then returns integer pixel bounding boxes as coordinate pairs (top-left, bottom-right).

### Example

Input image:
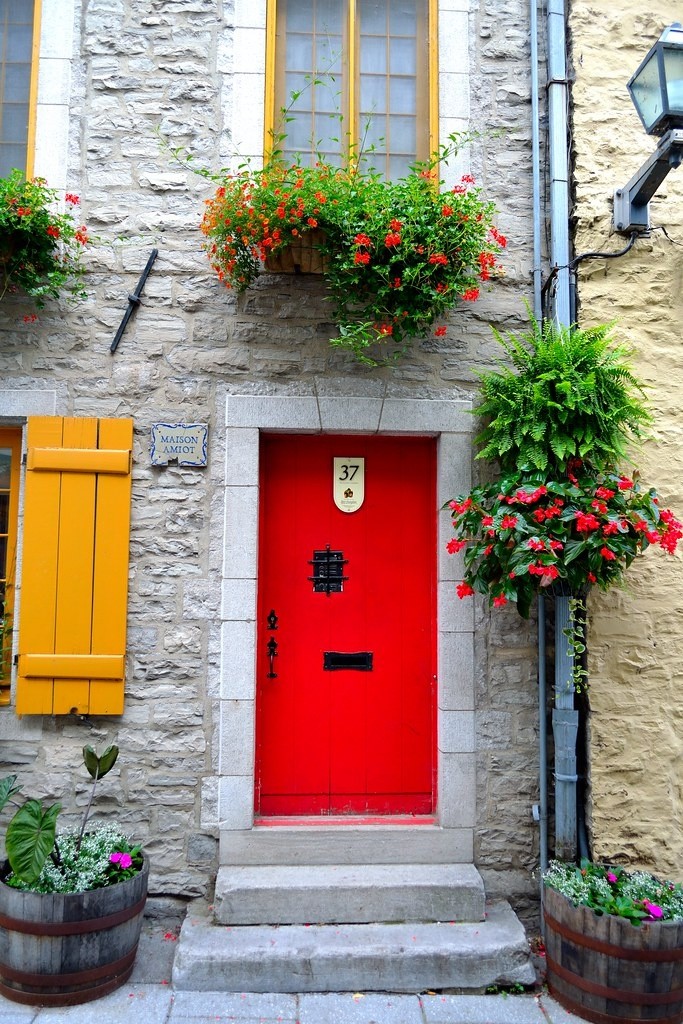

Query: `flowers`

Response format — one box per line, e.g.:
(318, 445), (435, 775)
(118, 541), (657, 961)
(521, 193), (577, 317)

(0, 745), (144, 895)
(155, 17), (508, 366)
(0, 167), (88, 325)
(531, 857), (683, 927)
(440, 460), (683, 605)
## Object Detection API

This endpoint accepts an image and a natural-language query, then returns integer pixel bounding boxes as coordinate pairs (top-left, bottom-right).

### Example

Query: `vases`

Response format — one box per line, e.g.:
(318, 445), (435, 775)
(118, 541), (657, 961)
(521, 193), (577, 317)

(543, 863), (683, 1024)
(264, 218), (340, 273)
(0, 843), (150, 1008)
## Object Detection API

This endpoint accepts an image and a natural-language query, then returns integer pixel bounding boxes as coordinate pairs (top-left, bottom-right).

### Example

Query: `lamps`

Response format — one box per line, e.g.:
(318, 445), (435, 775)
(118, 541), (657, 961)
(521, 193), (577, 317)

(612, 23), (683, 235)
